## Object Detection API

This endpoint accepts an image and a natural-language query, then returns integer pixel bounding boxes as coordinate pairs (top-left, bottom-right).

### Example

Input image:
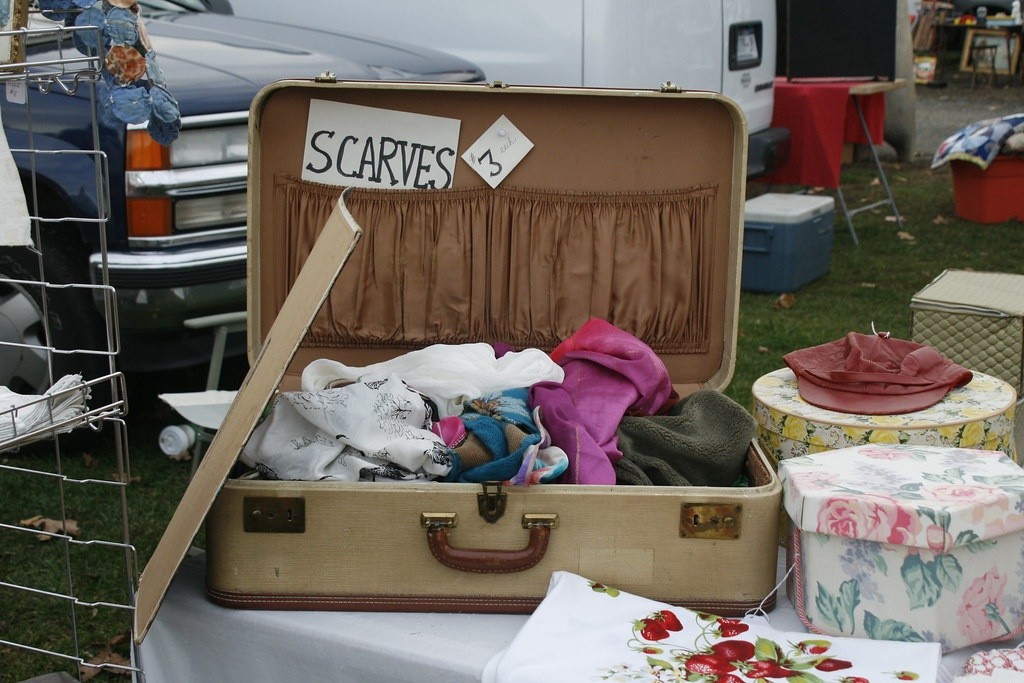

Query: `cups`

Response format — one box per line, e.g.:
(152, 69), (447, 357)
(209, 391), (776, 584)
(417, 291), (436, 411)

(159, 425), (196, 456)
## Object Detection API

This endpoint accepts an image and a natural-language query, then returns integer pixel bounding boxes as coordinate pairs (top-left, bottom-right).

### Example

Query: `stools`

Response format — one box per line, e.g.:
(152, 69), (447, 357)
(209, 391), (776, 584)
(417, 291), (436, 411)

(970, 44), (999, 88)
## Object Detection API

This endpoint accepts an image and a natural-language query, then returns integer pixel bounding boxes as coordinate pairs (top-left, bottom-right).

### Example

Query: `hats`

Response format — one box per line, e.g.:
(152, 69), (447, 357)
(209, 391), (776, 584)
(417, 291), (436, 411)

(782, 331), (974, 416)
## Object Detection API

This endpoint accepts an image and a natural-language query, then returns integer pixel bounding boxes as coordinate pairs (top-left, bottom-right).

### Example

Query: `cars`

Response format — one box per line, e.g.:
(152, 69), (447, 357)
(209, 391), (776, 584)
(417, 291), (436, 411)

(0, 0), (486, 459)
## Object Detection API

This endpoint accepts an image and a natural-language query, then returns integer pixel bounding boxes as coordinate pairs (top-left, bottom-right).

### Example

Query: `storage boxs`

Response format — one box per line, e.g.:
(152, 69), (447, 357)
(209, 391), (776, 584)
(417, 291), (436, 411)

(912, 267), (1024, 398)
(740, 194), (839, 295)
(953, 156), (1024, 223)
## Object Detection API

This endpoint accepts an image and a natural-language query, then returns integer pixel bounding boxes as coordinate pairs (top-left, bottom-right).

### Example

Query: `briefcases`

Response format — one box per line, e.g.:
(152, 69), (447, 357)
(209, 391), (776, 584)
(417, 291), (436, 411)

(207, 71), (786, 614)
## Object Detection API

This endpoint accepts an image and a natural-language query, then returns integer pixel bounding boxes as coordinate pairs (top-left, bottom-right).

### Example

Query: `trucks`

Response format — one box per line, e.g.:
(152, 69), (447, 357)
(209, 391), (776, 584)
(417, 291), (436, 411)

(136, 0), (791, 180)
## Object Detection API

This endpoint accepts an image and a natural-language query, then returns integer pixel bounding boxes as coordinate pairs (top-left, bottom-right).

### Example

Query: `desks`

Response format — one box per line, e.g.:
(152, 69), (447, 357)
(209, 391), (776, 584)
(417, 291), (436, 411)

(931, 19), (1024, 85)
(136, 546), (1024, 683)
(755, 77), (905, 244)
